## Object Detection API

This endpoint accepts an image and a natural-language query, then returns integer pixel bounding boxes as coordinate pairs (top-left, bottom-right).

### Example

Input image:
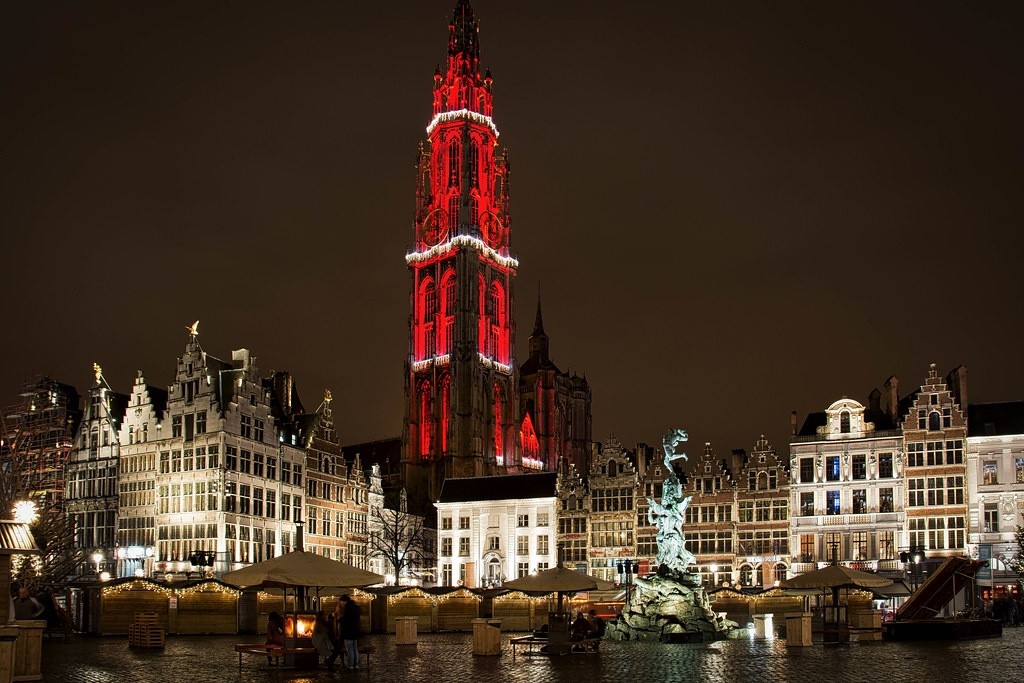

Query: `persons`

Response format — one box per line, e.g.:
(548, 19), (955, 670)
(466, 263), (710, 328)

(646, 495), (697, 572)
(662, 435), (689, 473)
(316, 594), (361, 672)
(265, 611), (284, 666)
(571, 610), (607, 653)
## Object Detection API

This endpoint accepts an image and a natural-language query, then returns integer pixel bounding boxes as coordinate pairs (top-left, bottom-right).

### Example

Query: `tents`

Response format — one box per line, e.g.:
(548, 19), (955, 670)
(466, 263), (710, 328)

(780, 565), (893, 604)
(502, 567), (614, 613)
(221, 549), (384, 613)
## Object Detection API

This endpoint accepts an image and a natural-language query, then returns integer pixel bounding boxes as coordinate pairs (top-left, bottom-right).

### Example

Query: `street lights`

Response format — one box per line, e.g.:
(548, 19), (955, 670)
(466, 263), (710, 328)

(899, 550), (925, 589)
(290, 432), (298, 556)
(211, 464), (232, 575)
(618, 555), (639, 604)
(91, 549), (102, 578)
(709, 562), (719, 591)
(277, 428), (287, 558)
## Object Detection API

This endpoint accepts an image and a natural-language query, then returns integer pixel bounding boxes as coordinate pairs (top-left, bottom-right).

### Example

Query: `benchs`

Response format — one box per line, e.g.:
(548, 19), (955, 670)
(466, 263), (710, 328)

(511, 630), (605, 663)
(234, 643), (377, 682)
